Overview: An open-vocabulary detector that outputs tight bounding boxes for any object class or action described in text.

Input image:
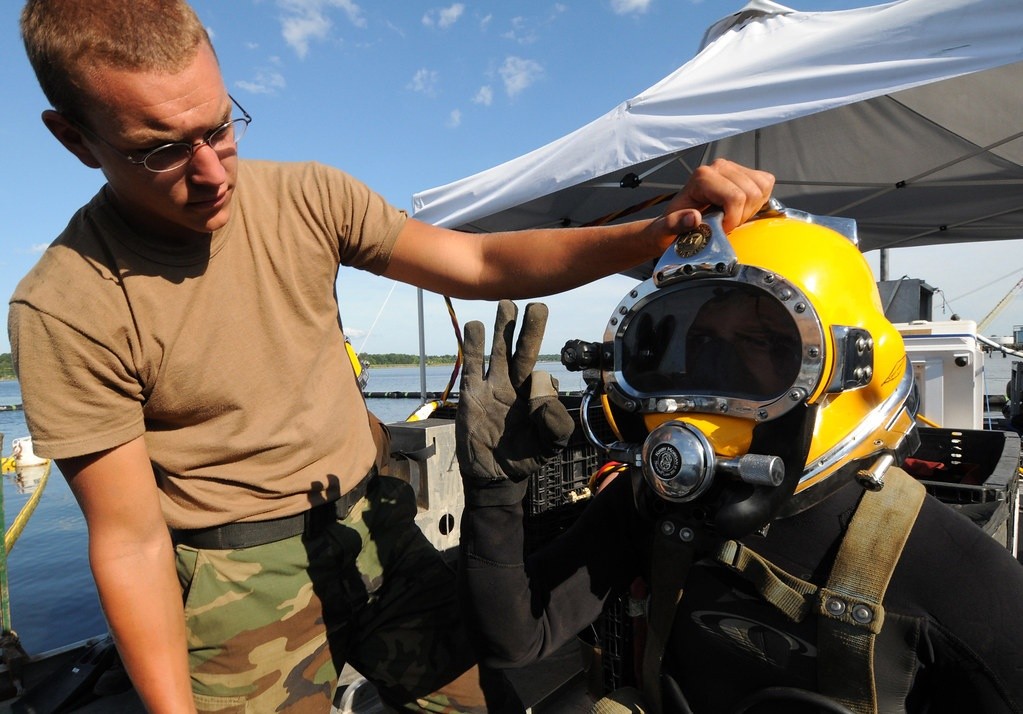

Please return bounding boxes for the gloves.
[456,299,575,508]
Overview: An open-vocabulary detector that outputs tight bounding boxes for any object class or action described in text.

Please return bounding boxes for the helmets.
[600,193,920,538]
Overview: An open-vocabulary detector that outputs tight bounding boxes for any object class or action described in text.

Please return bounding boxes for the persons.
[457,208,1023,714]
[14,454,23,482]
[8,0,778,714]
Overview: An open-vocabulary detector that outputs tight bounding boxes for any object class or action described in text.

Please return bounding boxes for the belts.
[170,465,377,550]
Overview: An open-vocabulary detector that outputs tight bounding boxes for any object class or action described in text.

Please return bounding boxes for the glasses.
[60,93,252,173]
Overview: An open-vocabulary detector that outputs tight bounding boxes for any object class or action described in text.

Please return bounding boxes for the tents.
[415,0,1023,476]
